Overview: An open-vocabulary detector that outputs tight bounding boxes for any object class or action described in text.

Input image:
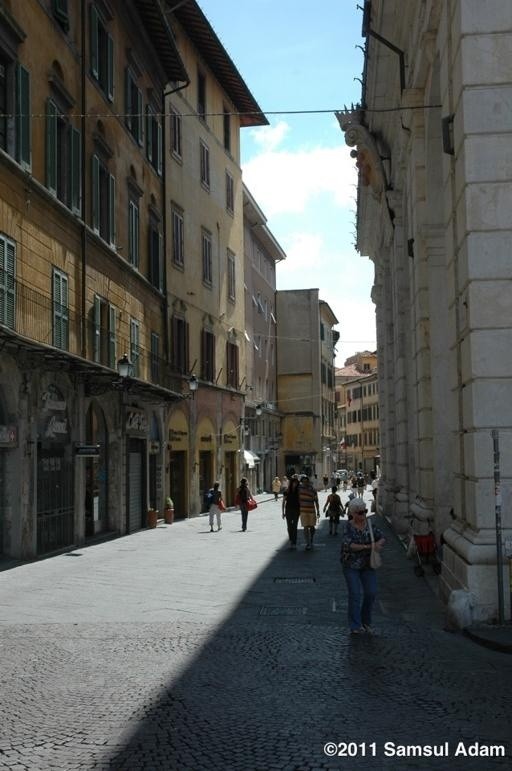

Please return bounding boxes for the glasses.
[355,508,368,516]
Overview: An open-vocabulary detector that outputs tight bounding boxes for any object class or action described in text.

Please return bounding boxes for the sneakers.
[354,625,380,637]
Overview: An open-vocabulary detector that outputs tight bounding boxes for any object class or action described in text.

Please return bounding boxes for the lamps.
[239,405,262,424]
[170,375,200,401]
[84,353,135,399]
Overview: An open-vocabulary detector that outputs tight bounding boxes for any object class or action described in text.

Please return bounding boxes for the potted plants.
[164,497,176,525]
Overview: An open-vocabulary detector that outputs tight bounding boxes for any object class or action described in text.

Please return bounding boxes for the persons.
[234,478,256,531]
[272,470,377,551]
[209,482,224,531]
[341,498,387,635]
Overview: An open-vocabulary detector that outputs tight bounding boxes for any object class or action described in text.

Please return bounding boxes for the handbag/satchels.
[218,499,225,510]
[204,493,211,501]
[370,550,383,569]
[246,499,257,511]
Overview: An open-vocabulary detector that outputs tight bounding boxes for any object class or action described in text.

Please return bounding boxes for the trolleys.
[410,517,443,569]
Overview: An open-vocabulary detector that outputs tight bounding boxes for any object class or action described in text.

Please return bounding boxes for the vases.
[148,509,159,529]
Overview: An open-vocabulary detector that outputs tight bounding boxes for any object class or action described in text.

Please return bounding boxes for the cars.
[334,468,350,481]
